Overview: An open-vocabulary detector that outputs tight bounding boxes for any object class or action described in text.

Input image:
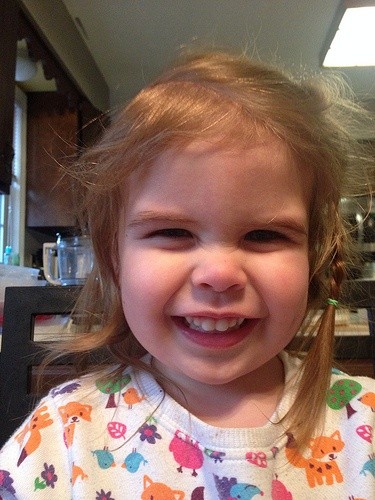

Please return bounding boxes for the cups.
[43,242,59,286]
[46,235,94,285]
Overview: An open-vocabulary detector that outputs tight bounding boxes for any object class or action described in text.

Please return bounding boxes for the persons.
[0,52,375,500]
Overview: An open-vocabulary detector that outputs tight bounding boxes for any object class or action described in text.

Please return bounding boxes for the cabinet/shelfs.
[26,84,99,236]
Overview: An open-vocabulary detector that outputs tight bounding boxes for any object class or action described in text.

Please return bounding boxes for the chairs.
[0,281,375,449]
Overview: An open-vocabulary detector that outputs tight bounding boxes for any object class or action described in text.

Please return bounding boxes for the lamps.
[317,0,375,69]
